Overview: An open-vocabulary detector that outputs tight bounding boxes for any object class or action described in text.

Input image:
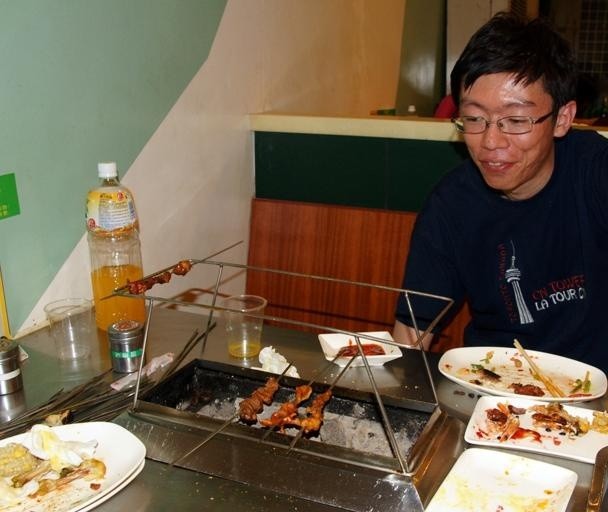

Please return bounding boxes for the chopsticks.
[512,338,567,399]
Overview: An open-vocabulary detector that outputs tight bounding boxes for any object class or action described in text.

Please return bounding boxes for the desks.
[1,303,608,511]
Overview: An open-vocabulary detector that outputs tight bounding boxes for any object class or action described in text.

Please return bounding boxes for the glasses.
[450,107,554,135]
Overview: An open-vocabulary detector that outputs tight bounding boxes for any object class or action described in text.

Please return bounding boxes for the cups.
[219,294,268,359]
[42,296,94,361]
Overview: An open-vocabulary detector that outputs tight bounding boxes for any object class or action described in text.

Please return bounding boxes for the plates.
[0,422,147,512]
[425,448,576,512]
[463,394,608,464]
[317,330,403,367]
[436,345,606,403]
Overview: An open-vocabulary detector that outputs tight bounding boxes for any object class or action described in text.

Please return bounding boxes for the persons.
[396,9,608,380]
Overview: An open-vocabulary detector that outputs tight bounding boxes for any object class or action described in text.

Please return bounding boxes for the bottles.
[106,318,146,374]
[0,339,24,397]
[84,160,147,334]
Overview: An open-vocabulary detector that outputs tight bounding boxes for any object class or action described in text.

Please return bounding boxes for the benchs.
[244,196,471,353]
